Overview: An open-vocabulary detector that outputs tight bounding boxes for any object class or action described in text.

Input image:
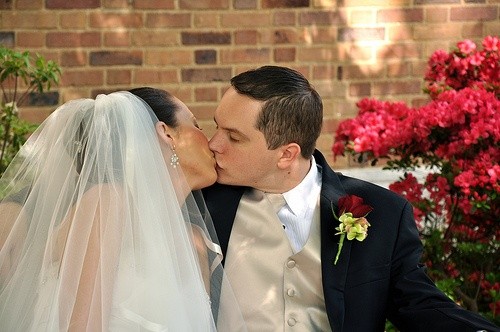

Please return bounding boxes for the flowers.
[330,194,374,267]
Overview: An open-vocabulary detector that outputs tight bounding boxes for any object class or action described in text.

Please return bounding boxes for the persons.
[0,86,218,332]
[1,65,500,332]
